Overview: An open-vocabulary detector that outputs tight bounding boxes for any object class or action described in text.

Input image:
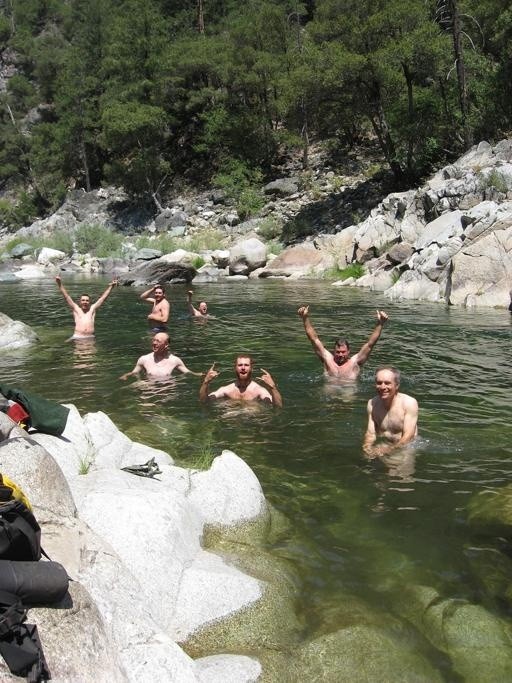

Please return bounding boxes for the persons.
[140,284,170,323]
[188,291,210,324]
[119,332,205,382]
[298,305,388,383]
[362,366,419,458]
[199,355,283,408]
[55,272,118,337]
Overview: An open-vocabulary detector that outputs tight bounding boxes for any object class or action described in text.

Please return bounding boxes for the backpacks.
[0,471,41,561]
